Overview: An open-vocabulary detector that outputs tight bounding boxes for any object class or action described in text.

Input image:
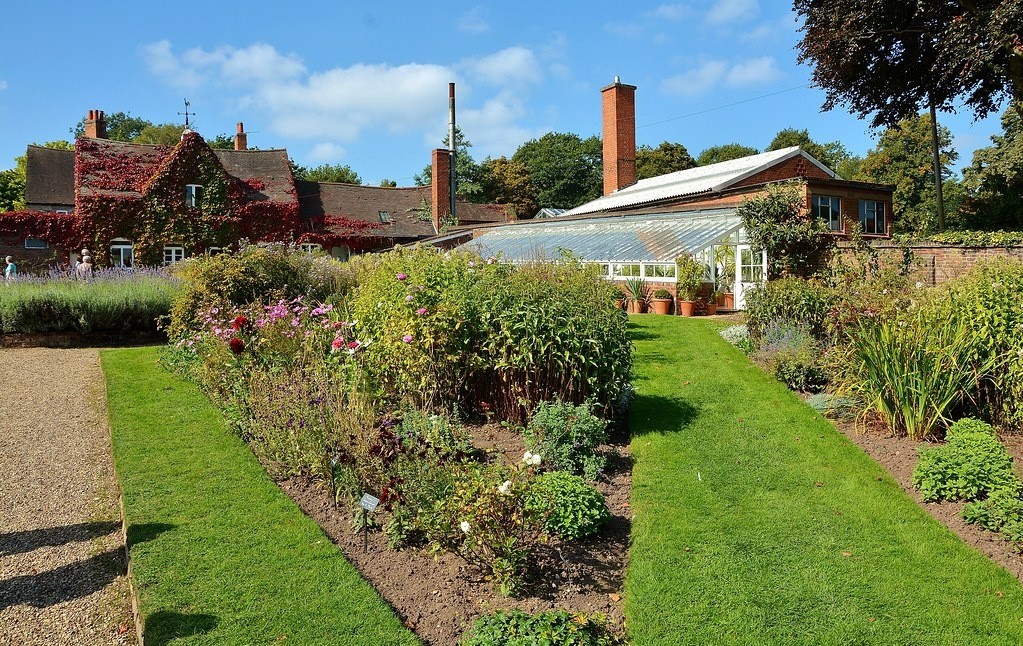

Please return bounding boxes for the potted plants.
[624,277,647,313]
[675,251,703,317]
[706,289,716,314]
[611,288,625,309]
[652,290,672,314]
[723,286,734,308]
[715,290,723,307]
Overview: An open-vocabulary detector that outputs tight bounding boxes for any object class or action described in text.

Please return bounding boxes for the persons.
[78,257,92,278]
[75,248,89,267]
[5,255,16,285]
